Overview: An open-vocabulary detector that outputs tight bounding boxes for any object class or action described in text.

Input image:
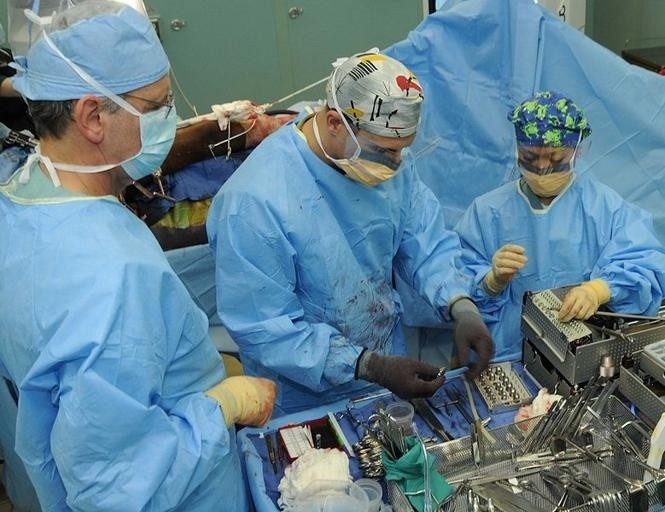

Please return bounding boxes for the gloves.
[447,295,494,380]
[354,347,447,401]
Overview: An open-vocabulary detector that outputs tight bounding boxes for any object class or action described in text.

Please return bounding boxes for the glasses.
[123,91,175,118]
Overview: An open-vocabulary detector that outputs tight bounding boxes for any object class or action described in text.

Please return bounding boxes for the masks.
[516,159,573,198]
[334,146,403,188]
[122,104,178,181]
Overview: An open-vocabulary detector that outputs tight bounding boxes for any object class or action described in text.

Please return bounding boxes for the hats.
[15,0,170,100]
[508,89,592,147]
[328,52,424,135]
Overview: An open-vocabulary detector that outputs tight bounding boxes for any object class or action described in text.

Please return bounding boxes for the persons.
[449,87,665,366]
[0,49,303,207]
[203,48,498,417]
[0,0,277,512]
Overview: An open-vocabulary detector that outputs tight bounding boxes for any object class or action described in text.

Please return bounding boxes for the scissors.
[335,399,369,438]
[351,436,383,478]
